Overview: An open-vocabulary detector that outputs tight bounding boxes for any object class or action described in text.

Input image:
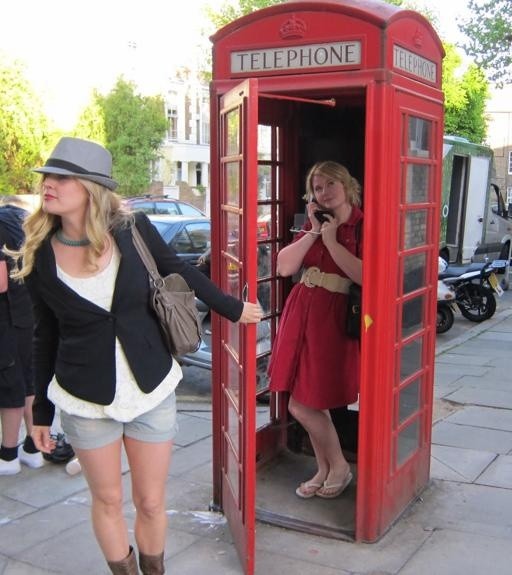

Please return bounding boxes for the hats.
[34,138,119,192]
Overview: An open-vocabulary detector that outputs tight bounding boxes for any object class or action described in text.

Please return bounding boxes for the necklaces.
[56,228,91,247]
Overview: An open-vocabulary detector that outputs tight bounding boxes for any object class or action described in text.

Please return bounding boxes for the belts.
[300,266,352,294]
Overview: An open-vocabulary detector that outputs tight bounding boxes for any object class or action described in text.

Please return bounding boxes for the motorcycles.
[163,247,278,405]
[438,255,509,322]
[403,255,457,334]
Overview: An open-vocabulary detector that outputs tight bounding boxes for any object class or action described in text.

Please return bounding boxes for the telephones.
[311,195,334,225]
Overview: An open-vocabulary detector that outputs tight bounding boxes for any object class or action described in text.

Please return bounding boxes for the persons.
[266,161,363,499]
[0,135,263,575]
[1,204,44,476]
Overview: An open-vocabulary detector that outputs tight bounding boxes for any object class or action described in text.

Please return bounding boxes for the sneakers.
[0,457,22,476]
[18,443,45,469]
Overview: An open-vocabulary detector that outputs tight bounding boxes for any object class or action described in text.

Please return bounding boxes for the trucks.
[404,132,512,274]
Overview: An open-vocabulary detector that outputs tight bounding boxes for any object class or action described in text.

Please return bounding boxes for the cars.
[141,213,274,309]
[121,196,208,222]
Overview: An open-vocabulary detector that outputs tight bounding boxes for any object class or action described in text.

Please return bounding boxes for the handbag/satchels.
[343,283,362,341]
[152,272,203,357]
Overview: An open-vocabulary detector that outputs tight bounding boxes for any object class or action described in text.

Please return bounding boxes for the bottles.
[65,459,81,476]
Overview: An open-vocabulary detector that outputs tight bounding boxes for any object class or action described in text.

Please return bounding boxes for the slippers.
[316,473,353,499]
[296,480,323,499]
